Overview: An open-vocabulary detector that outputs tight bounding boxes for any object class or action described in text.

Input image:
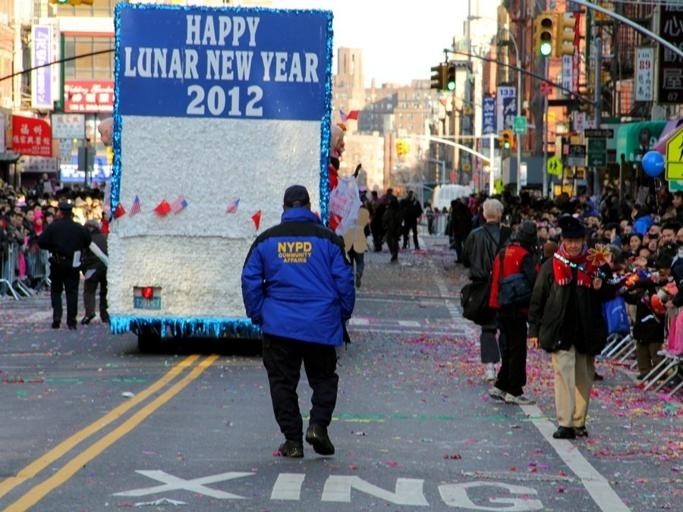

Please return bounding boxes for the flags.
[114,203,126,218]
[129,195,141,217]
[171,194,187,213]
[155,199,170,216]
[95,210,114,232]
[250,209,263,229]
[328,209,343,232]
[227,196,240,215]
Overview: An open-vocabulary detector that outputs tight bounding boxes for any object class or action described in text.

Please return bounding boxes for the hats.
[284,186,310,207]
[86,220,98,230]
[562,220,585,240]
[59,202,74,211]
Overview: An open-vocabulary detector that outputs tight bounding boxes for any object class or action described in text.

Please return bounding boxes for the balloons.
[642,150,665,176]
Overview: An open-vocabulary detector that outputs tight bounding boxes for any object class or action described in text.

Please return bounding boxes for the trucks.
[109,2,333,356]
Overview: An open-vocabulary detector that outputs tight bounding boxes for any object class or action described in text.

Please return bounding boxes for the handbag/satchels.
[496,274,529,305]
[605,299,631,333]
[460,280,496,323]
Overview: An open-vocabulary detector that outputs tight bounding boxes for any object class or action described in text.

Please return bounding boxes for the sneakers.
[486,368,496,380]
[68,323,77,330]
[100,311,110,323]
[505,393,536,405]
[305,424,334,455]
[280,440,304,458]
[575,426,589,437]
[553,426,577,440]
[81,311,95,324]
[52,322,60,329]
[488,387,506,400]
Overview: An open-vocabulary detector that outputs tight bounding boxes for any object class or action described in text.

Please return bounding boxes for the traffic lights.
[535,14,558,59]
[502,131,512,150]
[445,64,456,91]
[430,64,442,93]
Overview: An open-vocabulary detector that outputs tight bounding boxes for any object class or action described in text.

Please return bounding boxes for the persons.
[381,194,402,263]
[379,187,401,239]
[483,221,539,410]
[460,181,513,232]
[460,198,513,380]
[239,183,356,458]
[344,188,366,289]
[35,199,91,330]
[403,190,425,252]
[366,189,387,255]
[613,179,682,380]
[35,172,106,216]
[0,177,34,301]
[525,217,613,438]
[426,199,460,262]
[36,200,91,235]
[514,181,613,263]
[76,216,109,324]
